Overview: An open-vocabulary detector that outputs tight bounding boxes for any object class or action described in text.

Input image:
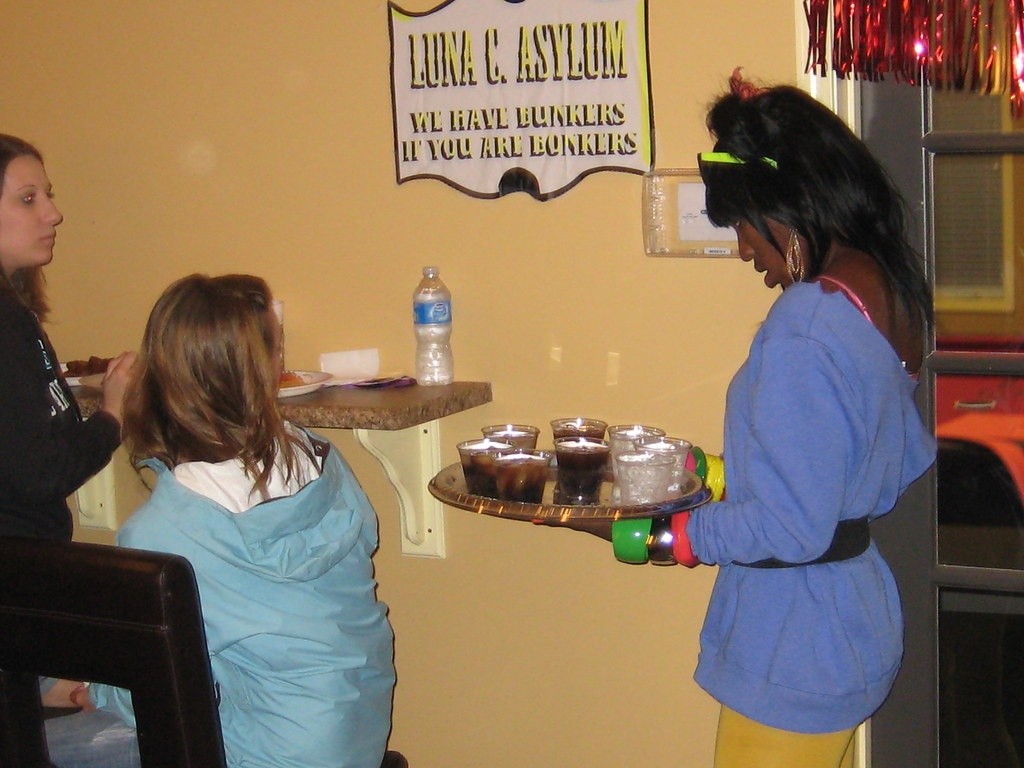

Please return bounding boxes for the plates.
[278,371,335,398]
[59,363,85,386]
[79,372,104,394]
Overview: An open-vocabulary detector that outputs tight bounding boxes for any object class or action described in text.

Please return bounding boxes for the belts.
[732,509,870,570]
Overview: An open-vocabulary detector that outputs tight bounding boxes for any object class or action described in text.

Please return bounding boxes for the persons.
[531,67,939,768]
[69,274,408,768]
[0,133,138,768]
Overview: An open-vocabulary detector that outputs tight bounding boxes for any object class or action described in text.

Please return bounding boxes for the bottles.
[412,266,455,386]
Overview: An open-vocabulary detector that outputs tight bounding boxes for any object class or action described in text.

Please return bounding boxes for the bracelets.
[648,518,679,566]
[671,510,701,568]
[612,518,652,564]
[685,446,725,503]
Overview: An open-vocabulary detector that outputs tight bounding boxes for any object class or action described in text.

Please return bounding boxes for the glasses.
[697,152,778,187]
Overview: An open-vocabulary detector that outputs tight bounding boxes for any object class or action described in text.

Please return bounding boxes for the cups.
[272,301,284,372]
[454,416,693,505]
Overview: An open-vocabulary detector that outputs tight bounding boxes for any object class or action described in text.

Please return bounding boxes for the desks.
[66,381,491,429]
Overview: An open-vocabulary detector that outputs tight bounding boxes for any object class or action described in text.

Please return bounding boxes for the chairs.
[0,535,413,768]
